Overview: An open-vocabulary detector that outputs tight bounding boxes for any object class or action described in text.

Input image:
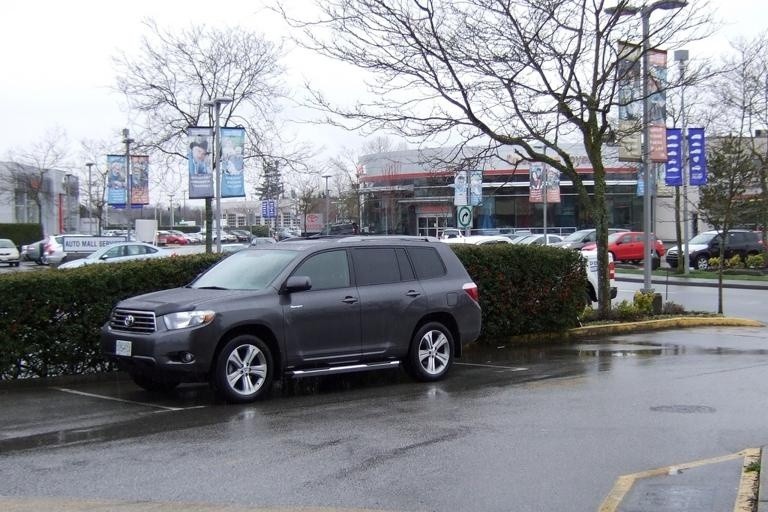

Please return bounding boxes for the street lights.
[168,195,175,228]
[65,173,72,233]
[181,190,187,221]
[203,96,234,252]
[604,0,688,317]
[673,49,690,275]
[85,163,94,235]
[122,128,134,241]
[321,175,332,236]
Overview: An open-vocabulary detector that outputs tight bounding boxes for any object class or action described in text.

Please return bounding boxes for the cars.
[440,229,631,263]
[56,242,173,270]
[360,226,377,234]
[552,196,614,226]
[21,226,298,254]
[0,239,20,266]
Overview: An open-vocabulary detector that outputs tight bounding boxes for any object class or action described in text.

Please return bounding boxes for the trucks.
[179,221,195,227]
[321,223,355,236]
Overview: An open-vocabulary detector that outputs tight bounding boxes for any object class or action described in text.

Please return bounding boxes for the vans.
[667,230,763,271]
[99,235,482,403]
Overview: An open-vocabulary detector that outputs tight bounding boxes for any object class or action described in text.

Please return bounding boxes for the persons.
[456,176,467,193]
[129,165,146,188]
[530,168,543,189]
[107,161,127,190]
[188,137,210,174]
[619,68,640,121]
[227,144,244,174]
[352,222,359,235]
[547,170,559,190]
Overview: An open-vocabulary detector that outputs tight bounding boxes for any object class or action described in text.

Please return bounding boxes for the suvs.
[39,234,93,265]
[581,231,665,266]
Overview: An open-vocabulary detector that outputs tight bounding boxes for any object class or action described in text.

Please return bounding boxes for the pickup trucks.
[439,234,618,316]
